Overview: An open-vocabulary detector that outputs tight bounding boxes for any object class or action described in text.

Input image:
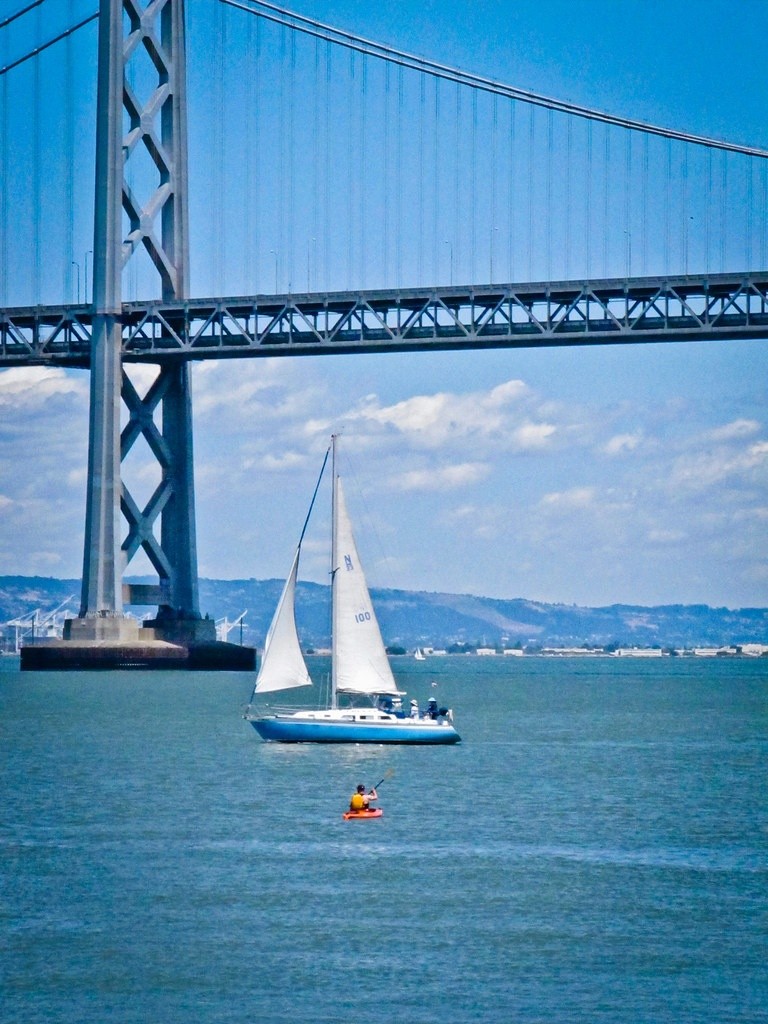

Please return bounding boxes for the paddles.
[349,767,394,807]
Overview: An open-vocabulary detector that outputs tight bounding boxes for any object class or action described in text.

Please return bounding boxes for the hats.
[428,697,435,701]
[410,700,417,705]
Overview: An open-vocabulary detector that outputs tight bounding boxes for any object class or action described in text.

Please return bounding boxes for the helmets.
[357,785,364,790]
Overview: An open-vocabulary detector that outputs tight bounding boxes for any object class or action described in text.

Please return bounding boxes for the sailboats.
[241,430,464,745]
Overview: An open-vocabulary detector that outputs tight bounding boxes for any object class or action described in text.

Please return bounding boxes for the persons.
[410,700,419,717]
[427,697,437,713]
[349,785,378,810]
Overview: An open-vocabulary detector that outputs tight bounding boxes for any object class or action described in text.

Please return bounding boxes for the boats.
[342,807,383,821]
